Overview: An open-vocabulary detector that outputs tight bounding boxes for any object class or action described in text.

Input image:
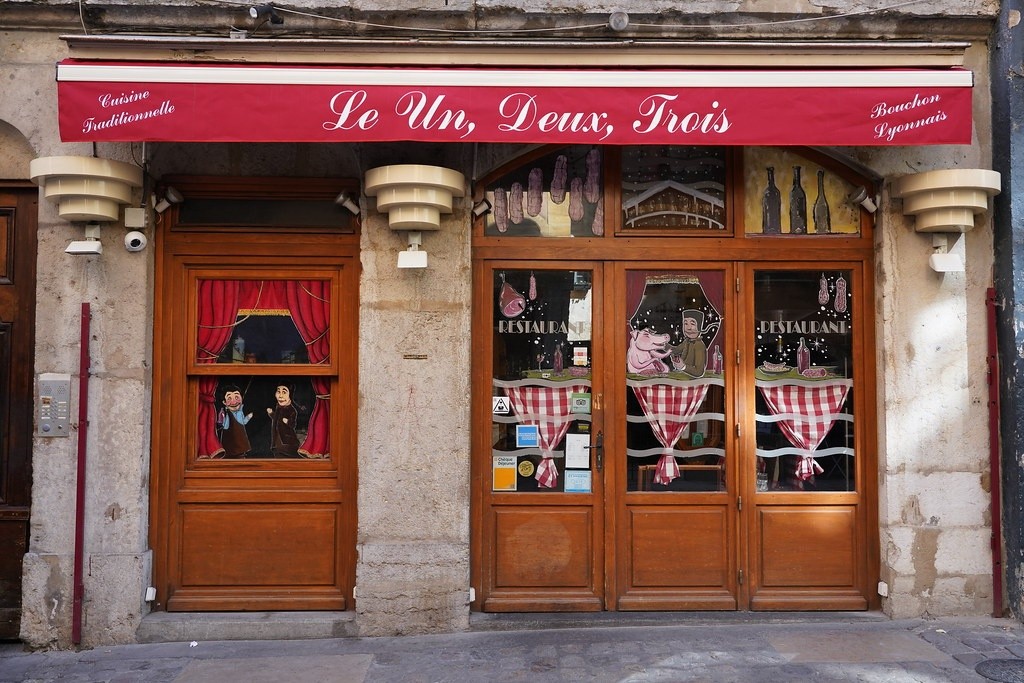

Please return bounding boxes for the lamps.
[853,185,877,215]
[154,187,183,215]
[335,191,359,216]
[250,3,284,24]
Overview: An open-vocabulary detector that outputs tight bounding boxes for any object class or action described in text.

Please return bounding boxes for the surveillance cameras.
[124,231,147,252]
